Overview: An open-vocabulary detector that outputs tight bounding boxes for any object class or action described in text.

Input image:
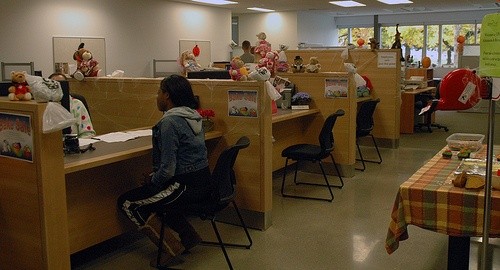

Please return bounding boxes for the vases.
[293,99,310,108]
[202,119,214,131]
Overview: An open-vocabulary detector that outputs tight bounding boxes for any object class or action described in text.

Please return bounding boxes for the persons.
[48,72,96,139]
[117,74,211,268]
[239,41,254,63]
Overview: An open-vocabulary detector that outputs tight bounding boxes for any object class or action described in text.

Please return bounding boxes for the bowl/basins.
[446,133,485,153]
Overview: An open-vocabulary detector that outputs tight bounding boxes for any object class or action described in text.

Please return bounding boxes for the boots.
[164,214,201,254]
[138,212,185,269]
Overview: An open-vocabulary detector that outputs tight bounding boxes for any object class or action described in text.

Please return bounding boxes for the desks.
[399,87,436,135]
[272,108,321,123]
[65,127,222,254]
[385,145,500,270]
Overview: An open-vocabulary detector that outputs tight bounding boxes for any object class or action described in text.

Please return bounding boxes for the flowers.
[198,109,215,120]
[292,92,311,103]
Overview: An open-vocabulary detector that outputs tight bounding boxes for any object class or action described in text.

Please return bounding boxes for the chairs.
[414,93,449,134]
[280,109,345,202]
[159,136,251,270]
[356,98,382,172]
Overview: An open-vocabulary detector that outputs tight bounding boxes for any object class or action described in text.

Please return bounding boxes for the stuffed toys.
[8,71,31,101]
[228,56,255,81]
[290,55,306,73]
[70,43,100,80]
[248,32,289,80]
[305,57,321,73]
[180,50,202,73]
[344,63,369,98]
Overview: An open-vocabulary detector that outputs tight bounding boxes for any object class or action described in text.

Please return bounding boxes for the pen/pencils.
[91,138,100,140]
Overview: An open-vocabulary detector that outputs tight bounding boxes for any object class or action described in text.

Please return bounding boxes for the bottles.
[64,134,79,153]
